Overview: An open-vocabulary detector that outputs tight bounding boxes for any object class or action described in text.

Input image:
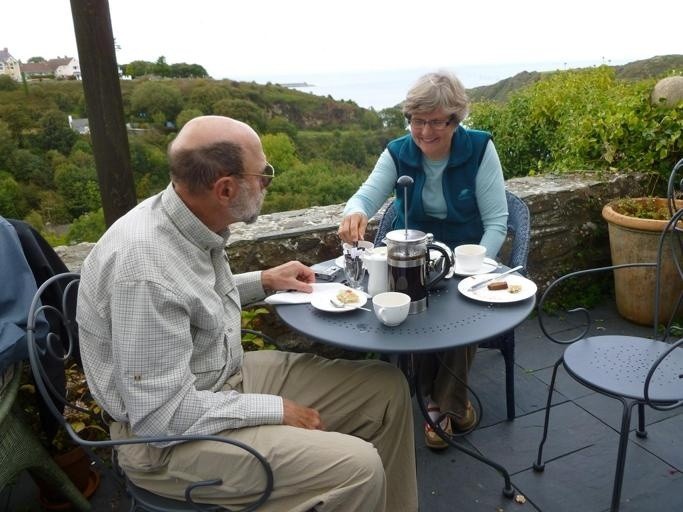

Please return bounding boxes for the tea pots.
[381,229,450,315]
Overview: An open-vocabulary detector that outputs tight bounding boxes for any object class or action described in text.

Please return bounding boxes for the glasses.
[408,117,454,131]
[227,162,274,188]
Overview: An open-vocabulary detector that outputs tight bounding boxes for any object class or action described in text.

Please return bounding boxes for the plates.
[451,257,499,276]
[309,287,367,314]
[430,241,450,262]
[457,273,538,304]
[335,256,366,271]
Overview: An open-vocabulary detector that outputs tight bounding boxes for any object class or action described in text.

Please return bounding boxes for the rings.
[338,227,342,230]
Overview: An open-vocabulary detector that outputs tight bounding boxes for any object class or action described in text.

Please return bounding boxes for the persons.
[335,71,509,452]
[73,113,420,511]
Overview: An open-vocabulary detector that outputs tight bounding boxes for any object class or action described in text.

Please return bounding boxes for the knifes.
[468,265,524,292]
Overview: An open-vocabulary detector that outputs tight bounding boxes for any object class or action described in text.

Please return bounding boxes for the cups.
[372,292,411,327]
[343,240,374,254]
[454,244,486,271]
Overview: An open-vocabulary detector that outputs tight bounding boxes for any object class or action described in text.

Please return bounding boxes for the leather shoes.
[452,400,477,432]
[424,399,453,450]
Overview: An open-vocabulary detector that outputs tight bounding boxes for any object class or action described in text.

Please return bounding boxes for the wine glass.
[343,261,364,292]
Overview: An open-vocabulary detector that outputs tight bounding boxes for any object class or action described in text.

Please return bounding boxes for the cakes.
[337,289,359,304]
